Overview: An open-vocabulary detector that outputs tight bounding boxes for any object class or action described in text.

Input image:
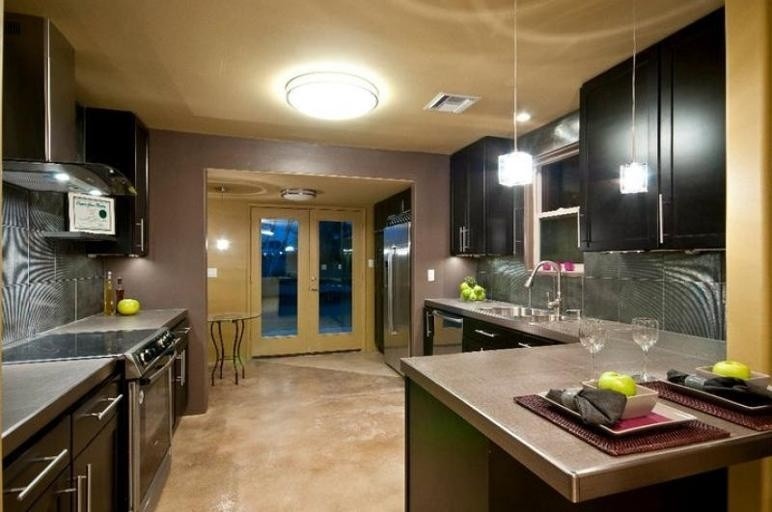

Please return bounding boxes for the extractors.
[3,11,138,199]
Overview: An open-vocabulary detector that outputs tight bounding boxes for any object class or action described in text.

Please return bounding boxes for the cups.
[631,317,659,382]
[579,316,606,382]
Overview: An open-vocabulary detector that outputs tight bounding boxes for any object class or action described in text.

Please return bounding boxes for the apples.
[598,371,636,398]
[712,360,749,382]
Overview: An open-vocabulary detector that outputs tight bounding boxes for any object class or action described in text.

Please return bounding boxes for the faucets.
[524,260,563,314]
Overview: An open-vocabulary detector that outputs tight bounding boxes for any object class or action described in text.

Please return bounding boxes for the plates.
[656,375,771,417]
[536,386,697,437]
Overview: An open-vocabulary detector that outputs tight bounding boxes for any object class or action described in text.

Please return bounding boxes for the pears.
[460,283,487,302]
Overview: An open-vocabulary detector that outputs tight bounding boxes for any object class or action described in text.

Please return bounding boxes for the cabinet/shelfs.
[579,4,726,254]
[65,109,149,258]
[423,297,464,358]
[399,339,772,512]
[89,307,194,437]
[450,135,524,258]
[2,357,125,512]
[518,317,635,348]
[463,303,521,356]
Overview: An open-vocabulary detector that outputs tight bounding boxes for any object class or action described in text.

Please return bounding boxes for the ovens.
[126,349,177,512]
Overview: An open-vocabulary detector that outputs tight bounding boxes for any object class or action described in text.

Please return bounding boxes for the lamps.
[284,71,379,120]
[619,3,648,194]
[280,188,317,201]
[498,0,533,187]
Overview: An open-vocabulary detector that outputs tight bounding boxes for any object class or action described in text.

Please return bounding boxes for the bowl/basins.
[581,380,658,420]
[694,364,770,390]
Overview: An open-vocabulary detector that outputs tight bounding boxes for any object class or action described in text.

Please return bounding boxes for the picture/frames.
[68,192,116,235]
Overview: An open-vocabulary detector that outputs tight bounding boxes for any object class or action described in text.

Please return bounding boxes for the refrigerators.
[379,222,412,380]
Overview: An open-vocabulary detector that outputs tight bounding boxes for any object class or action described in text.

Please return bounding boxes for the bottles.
[115,277,124,313]
[104,271,116,316]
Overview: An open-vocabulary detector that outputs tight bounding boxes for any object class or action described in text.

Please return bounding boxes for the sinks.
[513,316,572,327]
[481,305,551,317]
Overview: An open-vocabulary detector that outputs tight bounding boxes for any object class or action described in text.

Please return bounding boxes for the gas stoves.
[3,326,181,380]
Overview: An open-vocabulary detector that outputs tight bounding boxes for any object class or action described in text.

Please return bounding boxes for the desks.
[208,311,262,386]
